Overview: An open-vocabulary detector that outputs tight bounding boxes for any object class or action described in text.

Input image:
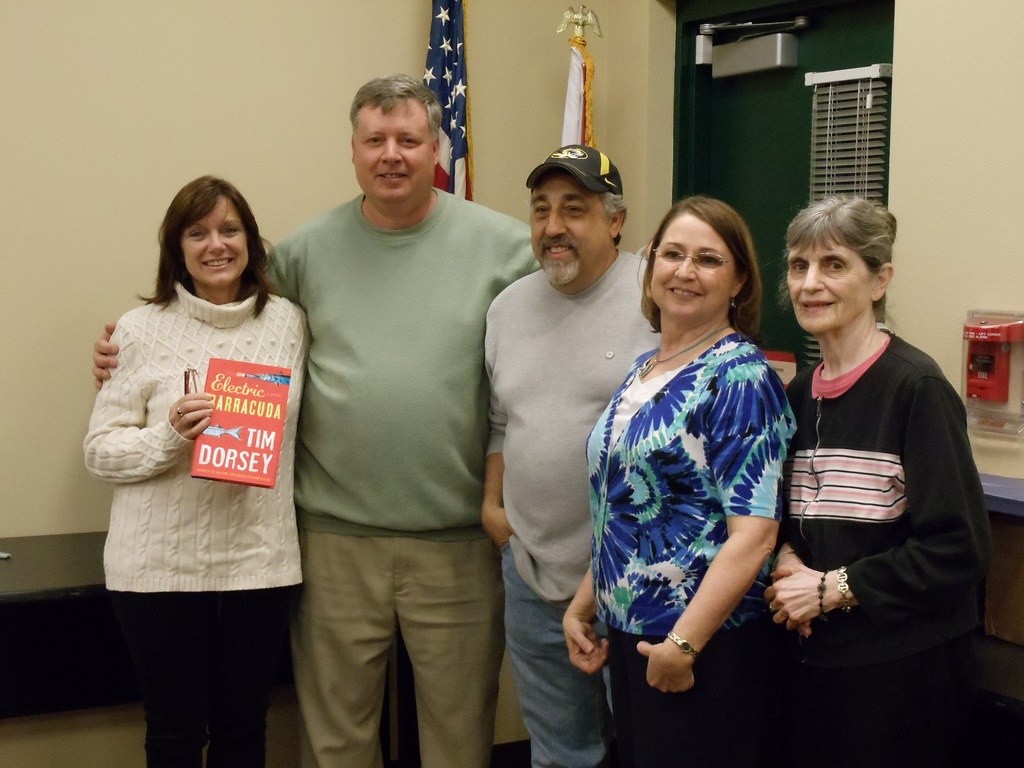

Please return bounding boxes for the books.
[191,358,292,489]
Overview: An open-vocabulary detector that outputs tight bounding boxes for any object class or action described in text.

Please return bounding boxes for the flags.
[561,38,597,150]
[419,0,475,206]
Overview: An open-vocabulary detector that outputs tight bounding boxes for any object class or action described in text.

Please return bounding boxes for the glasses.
[651,249,736,269]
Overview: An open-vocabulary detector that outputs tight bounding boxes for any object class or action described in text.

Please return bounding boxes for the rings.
[770,603,775,613]
[177,408,184,416]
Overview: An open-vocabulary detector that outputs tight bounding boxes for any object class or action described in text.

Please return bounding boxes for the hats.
[525,144,623,201]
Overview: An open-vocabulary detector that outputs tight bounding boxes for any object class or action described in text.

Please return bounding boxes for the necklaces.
[639,326,728,378]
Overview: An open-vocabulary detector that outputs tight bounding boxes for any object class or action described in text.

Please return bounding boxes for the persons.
[478,146,663,768]
[94,72,544,768]
[83,175,314,768]
[763,197,995,768]
[562,195,797,768]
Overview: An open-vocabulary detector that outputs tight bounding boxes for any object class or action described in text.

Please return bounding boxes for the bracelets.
[837,566,851,612]
[773,550,794,566]
[817,571,828,621]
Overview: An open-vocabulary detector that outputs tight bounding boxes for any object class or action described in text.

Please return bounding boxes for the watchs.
[667,631,699,658]
